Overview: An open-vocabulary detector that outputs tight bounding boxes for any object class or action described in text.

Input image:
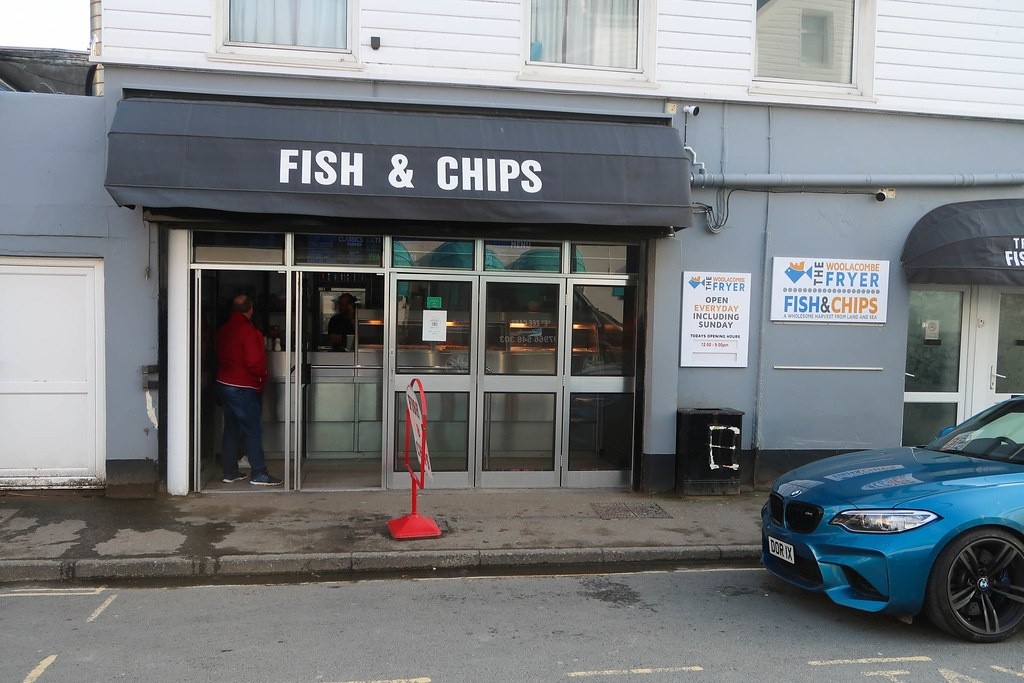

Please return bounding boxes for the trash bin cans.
[676,408,745,496]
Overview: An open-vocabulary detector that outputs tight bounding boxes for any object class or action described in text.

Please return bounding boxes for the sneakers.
[249,473,283,486]
[222,473,248,483]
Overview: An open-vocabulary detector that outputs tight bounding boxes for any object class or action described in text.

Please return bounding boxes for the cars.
[761,394,1024,644]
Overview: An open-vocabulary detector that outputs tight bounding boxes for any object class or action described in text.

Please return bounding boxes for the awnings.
[105,85,694,237]
[902,196,1024,287]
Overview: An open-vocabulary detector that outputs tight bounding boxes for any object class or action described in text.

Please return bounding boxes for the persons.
[326,293,357,343]
[214,295,288,485]
